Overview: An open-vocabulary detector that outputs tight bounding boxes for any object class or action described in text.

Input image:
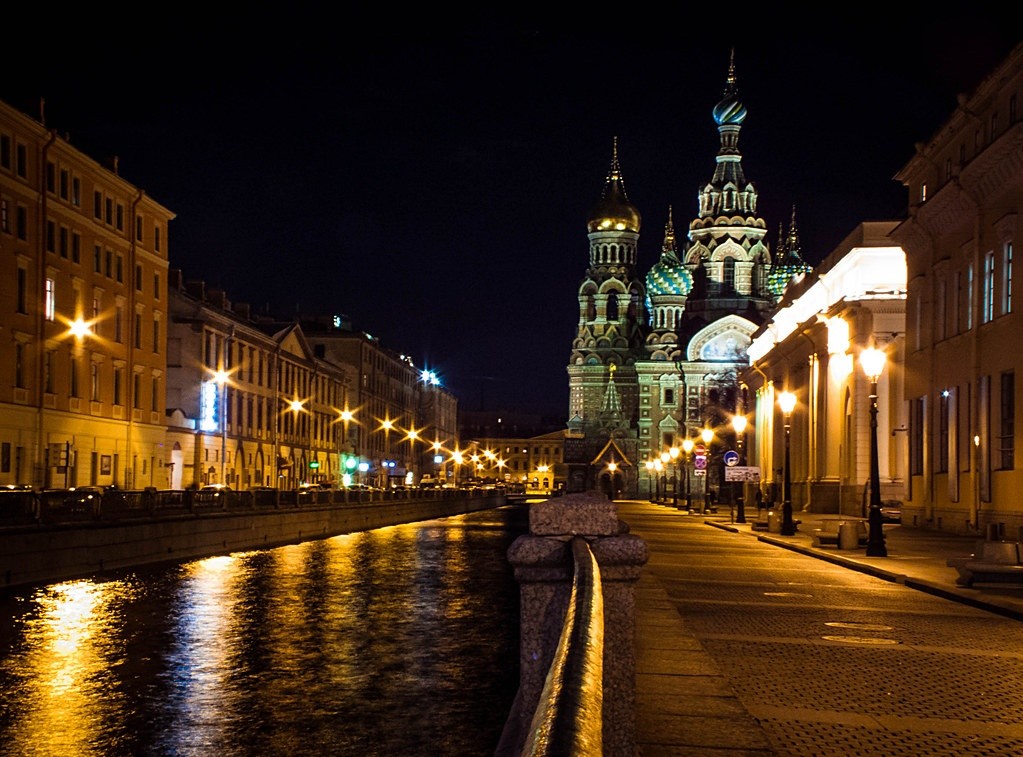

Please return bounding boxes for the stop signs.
[695,445,706,456]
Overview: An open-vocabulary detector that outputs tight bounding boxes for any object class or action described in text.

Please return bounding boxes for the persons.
[755,489,771,511]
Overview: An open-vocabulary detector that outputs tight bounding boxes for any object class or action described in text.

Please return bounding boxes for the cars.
[199,485,238,505]
[65,485,103,512]
[299,478,496,498]
[881,498,903,522]
[0,485,40,520]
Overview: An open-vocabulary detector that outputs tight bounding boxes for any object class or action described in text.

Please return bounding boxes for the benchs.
[807,529,869,549]
[748,519,801,531]
[946,556,1023,587]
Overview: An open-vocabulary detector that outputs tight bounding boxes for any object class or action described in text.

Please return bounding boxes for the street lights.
[646,461,654,502]
[652,459,662,504]
[777,391,799,535]
[669,447,679,507]
[661,452,671,505]
[732,415,748,523]
[700,429,714,514]
[858,346,893,560]
[683,440,695,511]
